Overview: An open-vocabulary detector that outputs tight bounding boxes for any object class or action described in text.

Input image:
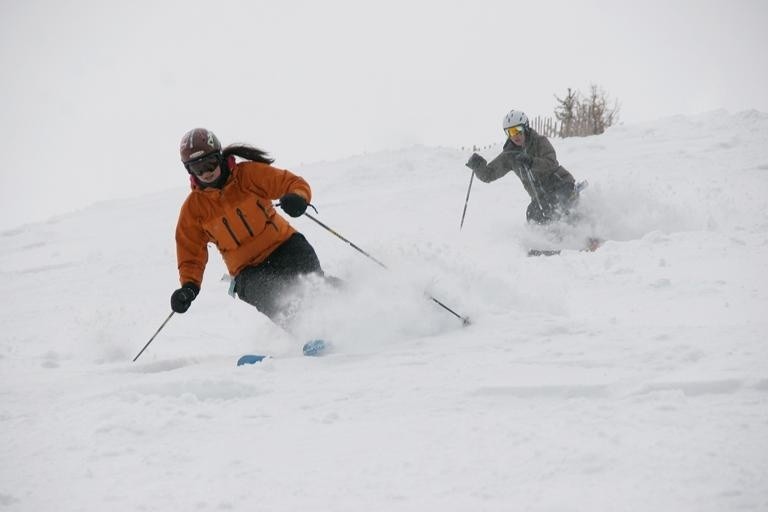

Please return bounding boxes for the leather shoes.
[465,153,487,174]
[171,282,200,313]
[515,151,532,168]
[279,192,307,217]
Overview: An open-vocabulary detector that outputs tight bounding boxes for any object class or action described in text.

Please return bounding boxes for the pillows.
[503,110,529,129]
[180,128,221,164]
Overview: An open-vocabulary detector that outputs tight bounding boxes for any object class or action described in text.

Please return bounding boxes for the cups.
[187,155,222,176]
[505,126,526,136]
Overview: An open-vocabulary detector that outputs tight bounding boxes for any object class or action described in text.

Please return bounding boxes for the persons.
[465,111,575,256]
[170,129,325,336]
